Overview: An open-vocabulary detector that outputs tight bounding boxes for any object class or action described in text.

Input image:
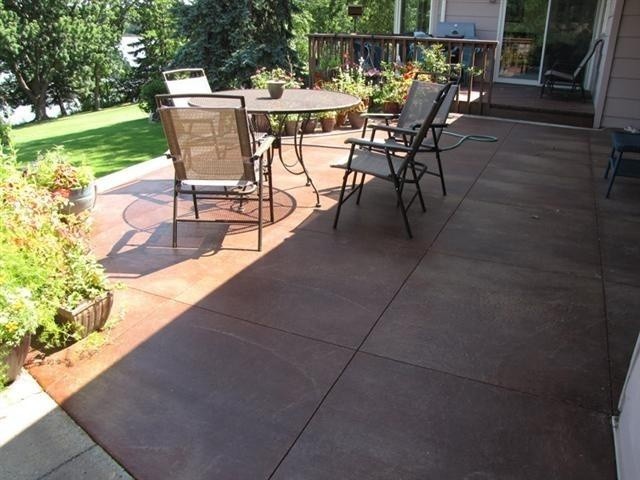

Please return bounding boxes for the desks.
[188,90,361,212]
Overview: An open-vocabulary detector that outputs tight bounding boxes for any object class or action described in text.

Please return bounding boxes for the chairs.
[353,22,476,87]
[540,39,602,103]
[155,94,275,251]
[330,82,455,238]
[604,131,640,198]
[352,80,458,196]
[161,68,268,195]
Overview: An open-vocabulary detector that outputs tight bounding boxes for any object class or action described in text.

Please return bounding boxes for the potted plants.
[373,61,404,113]
[16,225,128,350]
[284,114,303,136]
[0,261,40,385]
[352,83,376,106]
[335,109,350,127]
[348,101,367,128]
[301,113,318,133]
[315,111,337,132]
[25,144,98,225]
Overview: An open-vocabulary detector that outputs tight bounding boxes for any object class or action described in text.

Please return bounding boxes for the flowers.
[249,66,305,90]
[403,61,430,106]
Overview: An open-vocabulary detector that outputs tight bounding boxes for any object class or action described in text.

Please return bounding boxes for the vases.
[253,113,272,135]
